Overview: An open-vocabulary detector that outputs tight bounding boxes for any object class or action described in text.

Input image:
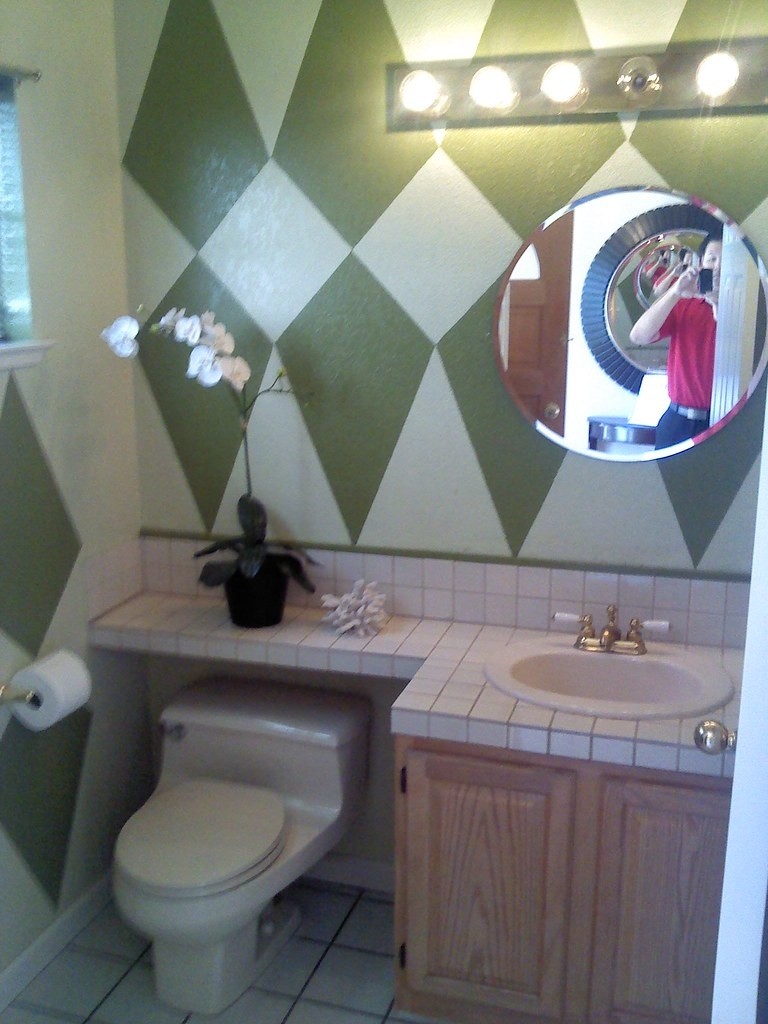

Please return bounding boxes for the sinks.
[482,635,737,720]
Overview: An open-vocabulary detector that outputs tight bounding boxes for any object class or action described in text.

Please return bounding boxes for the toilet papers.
[6,646,93,733]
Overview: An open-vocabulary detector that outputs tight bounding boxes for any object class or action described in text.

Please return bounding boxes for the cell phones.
[699,269,713,294]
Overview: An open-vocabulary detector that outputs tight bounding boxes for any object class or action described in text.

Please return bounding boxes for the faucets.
[551,605,673,656]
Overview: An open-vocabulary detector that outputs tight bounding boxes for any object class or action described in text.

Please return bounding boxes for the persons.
[628,230,722,452]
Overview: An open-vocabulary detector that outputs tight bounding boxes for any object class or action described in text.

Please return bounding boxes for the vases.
[228,564,289,628]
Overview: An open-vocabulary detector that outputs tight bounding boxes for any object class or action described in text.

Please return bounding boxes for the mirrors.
[491,185,768,463]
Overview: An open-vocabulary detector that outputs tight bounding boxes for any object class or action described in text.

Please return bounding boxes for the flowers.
[99,305,323,597]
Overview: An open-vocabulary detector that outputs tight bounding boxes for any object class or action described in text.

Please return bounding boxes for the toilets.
[110,679,374,1014]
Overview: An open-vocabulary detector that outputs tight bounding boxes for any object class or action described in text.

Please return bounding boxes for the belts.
[669,399,710,422]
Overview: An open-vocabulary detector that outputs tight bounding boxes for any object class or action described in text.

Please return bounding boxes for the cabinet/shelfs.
[391,733,732,1024]
